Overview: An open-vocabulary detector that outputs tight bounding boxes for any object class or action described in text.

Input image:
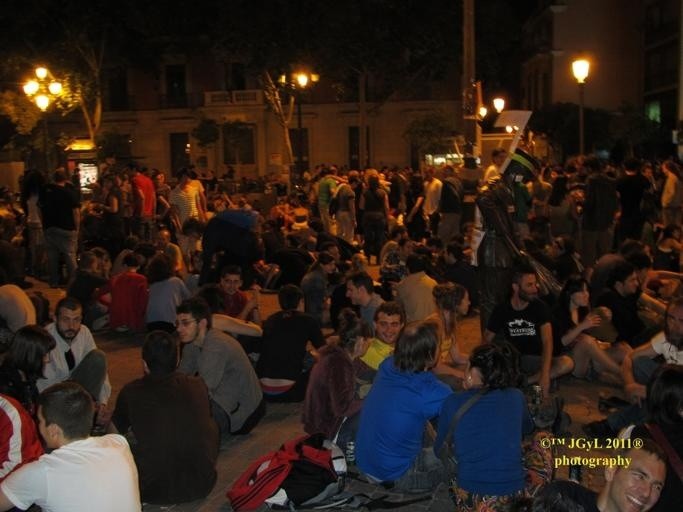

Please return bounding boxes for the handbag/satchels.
[440,443,458,475]
[328,199,340,216]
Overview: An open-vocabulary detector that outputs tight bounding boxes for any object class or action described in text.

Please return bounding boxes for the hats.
[176,167,191,181]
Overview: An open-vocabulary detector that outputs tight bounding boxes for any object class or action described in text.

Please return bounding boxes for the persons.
[0,145,683,403]
[622,363,683,512]
[433,340,557,512]
[353,321,447,502]
[0,381,143,512]
[301,308,365,442]
[105,330,221,503]
[533,438,669,512]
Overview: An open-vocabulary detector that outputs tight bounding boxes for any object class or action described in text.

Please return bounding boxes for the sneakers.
[265,264,282,289]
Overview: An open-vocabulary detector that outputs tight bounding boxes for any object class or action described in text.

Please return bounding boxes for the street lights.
[569,57,590,158]
[23,66,62,178]
[278,71,319,178]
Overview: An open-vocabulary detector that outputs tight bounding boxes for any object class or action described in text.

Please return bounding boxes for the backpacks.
[224,433,347,512]
